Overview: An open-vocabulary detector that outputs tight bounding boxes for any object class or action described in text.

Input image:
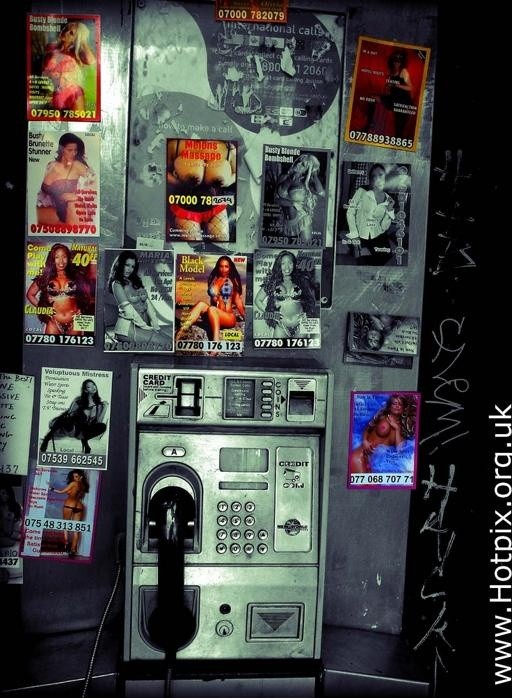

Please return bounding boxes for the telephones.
[148,486,196,648]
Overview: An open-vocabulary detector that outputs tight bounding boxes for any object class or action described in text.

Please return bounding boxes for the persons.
[351,395,416,472]
[41,23,96,118]
[39,133,97,225]
[361,46,415,139]
[46,470,86,556]
[166,140,236,241]
[273,154,327,246]
[346,166,397,265]
[254,250,319,340]
[106,253,171,346]
[0,486,23,583]
[179,257,244,355]
[40,379,106,455]
[355,314,392,352]
[27,244,93,336]
